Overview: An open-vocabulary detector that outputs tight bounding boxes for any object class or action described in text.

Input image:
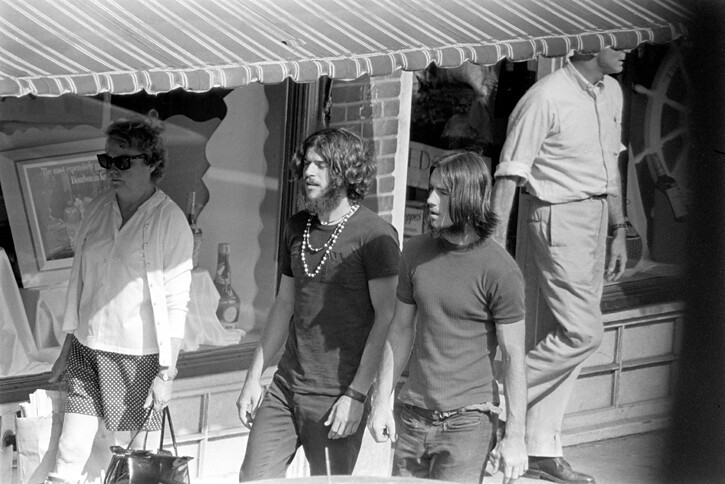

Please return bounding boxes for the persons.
[367,149,530,484]
[236,129,403,484]
[44,119,194,484]
[486,47,631,484]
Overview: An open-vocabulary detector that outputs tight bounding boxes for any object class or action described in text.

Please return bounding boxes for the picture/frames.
[0,137,108,288]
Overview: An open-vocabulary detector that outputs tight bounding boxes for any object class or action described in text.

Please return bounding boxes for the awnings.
[0,0,697,99]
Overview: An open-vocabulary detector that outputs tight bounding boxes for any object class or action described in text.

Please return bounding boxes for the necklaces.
[301,200,358,278]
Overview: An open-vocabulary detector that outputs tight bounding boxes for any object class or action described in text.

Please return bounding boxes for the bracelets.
[344,387,366,403]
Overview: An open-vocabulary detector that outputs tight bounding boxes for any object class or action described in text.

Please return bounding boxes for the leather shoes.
[522,454,597,484]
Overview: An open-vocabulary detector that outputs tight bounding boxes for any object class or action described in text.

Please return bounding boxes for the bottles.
[184,191,202,271]
[212,243,241,330]
[64,189,82,254]
[649,152,689,221]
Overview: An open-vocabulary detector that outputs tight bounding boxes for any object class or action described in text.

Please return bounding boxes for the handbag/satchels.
[14,388,116,484]
[103,403,195,484]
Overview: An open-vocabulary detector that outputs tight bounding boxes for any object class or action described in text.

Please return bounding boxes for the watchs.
[611,223,628,231]
[157,373,175,381]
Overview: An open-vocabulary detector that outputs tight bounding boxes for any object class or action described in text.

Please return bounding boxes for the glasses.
[96,153,146,170]
